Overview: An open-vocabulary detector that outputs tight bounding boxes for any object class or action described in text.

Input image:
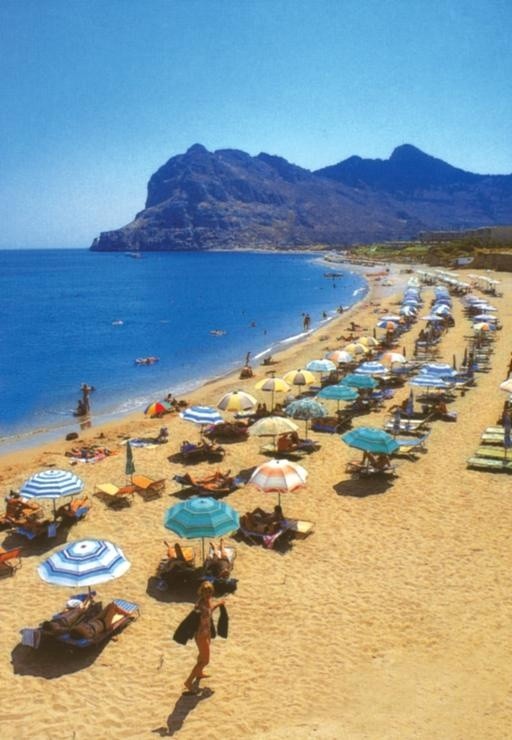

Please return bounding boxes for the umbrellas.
[125,440,138,490]
[16,469,85,517]
[163,495,243,568]
[36,537,133,604]
[245,458,310,506]
[143,400,172,419]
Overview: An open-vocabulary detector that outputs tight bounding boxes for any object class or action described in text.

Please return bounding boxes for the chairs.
[162,541,248,590]
[172,437,243,500]
[259,435,322,462]
[341,408,439,481]
[93,480,138,509]
[237,505,317,551]
[0,495,92,577]
[129,473,167,502]
[18,590,146,649]
[467,424,511,473]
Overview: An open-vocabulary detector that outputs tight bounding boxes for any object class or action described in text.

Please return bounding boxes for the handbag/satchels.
[173,610,200,645]
[190,617,216,639]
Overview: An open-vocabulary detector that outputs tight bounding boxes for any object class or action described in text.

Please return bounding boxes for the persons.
[72,399,88,417]
[201,467,232,493]
[9,490,40,512]
[161,540,191,592]
[40,589,97,630]
[65,443,112,460]
[134,356,161,365]
[237,512,280,535]
[182,580,228,692]
[246,503,286,523]
[52,496,90,521]
[165,393,188,413]
[303,304,344,330]
[23,516,52,531]
[68,601,134,640]
[79,383,95,411]
[208,537,231,579]
